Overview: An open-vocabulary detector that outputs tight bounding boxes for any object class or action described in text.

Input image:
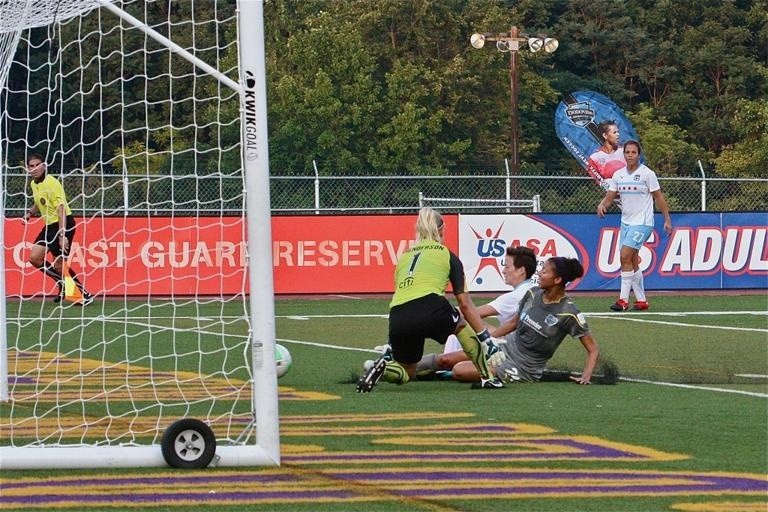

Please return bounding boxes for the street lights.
[470,26,560,213]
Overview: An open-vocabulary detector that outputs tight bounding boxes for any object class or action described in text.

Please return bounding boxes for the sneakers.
[610,299,648,311]
[54,286,65,302]
[479,377,504,388]
[82,292,91,303]
[356,344,393,394]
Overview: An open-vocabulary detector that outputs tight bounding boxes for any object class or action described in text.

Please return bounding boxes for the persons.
[366,246,541,379]
[25,157,93,308]
[418,257,601,384]
[597,140,670,311]
[356,203,508,393]
[587,119,630,183]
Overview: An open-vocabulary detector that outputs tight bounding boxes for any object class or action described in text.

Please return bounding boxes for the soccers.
[276,344,292,378]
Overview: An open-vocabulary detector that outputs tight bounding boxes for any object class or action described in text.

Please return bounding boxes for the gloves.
[476,329,506,367]
